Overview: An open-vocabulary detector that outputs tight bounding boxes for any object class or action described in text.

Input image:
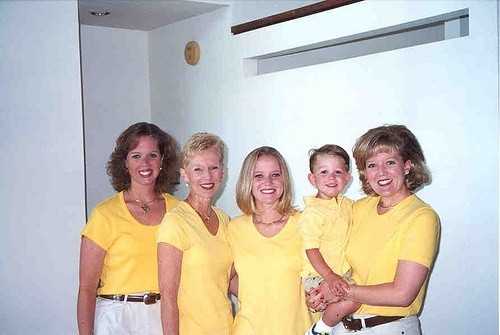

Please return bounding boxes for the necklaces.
[185,197,216,223]
[377,193,413,209]
[252,211,285,225]
[127,190,158,213]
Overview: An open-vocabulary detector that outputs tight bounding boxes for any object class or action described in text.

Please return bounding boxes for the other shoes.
[305,323,332,335]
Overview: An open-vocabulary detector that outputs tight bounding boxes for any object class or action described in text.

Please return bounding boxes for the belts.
[96,291,160,305]
[341,315,405,332]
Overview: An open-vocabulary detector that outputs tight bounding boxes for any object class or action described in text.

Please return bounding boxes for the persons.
[299,143,361,335]
[76,121,181,335]
[305,121,441,335]
[228,146,315,335]
[157,128,239,335]
